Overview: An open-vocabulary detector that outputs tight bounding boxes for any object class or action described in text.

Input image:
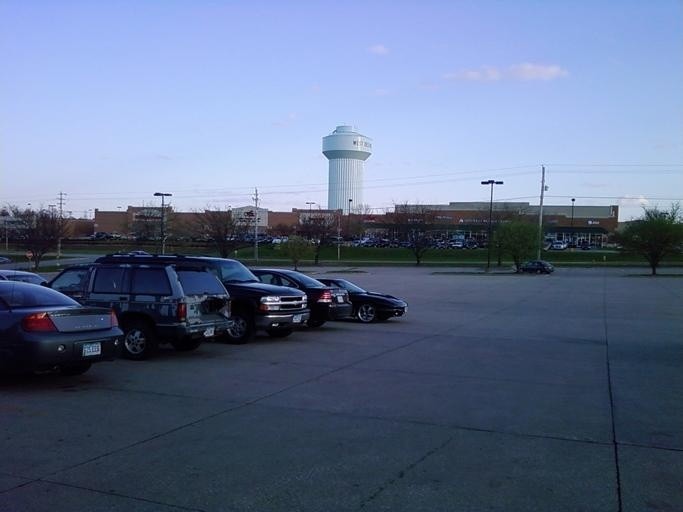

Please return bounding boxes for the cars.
[518,260,554,274]
[0,268,126,388]
[90,231,237,241]
[0,257,12,263]
[246,233,494,249]
[582,242,591,250]
[250,266,409,330]
[552,240,569,250]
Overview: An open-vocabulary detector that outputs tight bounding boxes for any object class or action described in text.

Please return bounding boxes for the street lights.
[306,202,316,226]
[153,192,172,254]
[481,179,504,269]
[348,199,353,232]
[571,198,575,225]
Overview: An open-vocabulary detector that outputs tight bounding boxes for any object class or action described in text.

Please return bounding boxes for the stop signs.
[25,250,33,260]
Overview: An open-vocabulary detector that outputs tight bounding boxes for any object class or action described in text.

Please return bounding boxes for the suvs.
[40,249,313,362]
